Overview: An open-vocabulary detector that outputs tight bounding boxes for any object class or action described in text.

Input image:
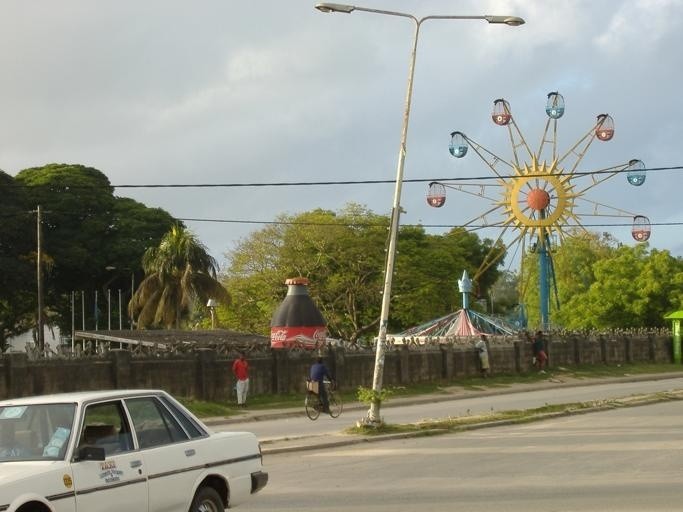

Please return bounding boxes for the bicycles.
[304,376,343,420]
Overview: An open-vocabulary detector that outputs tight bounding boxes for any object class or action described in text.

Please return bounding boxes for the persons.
[232,351,250,408]
[310,357,335,413]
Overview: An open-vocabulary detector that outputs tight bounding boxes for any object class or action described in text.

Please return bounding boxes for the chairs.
[1,420,165,458]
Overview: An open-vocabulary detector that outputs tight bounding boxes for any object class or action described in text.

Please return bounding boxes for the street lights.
[104,267,135,330]
[205,297,217,328]
[313,1,526,425]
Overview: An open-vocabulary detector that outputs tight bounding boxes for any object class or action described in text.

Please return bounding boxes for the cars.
[0,390,269,512]
